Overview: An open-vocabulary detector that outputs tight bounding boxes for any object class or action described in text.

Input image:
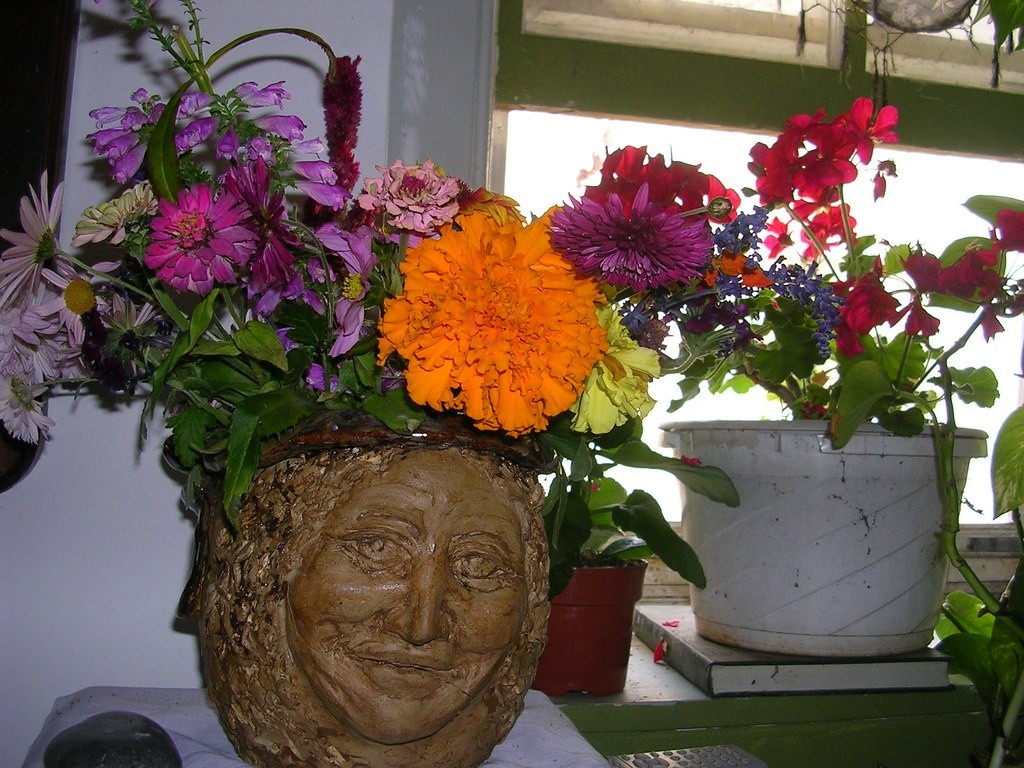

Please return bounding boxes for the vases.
[523,558,649,696]
[659,420,989,663]
[156,396,554,768]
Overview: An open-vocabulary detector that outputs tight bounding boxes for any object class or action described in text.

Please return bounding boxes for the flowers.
[0,0,1024,461]
